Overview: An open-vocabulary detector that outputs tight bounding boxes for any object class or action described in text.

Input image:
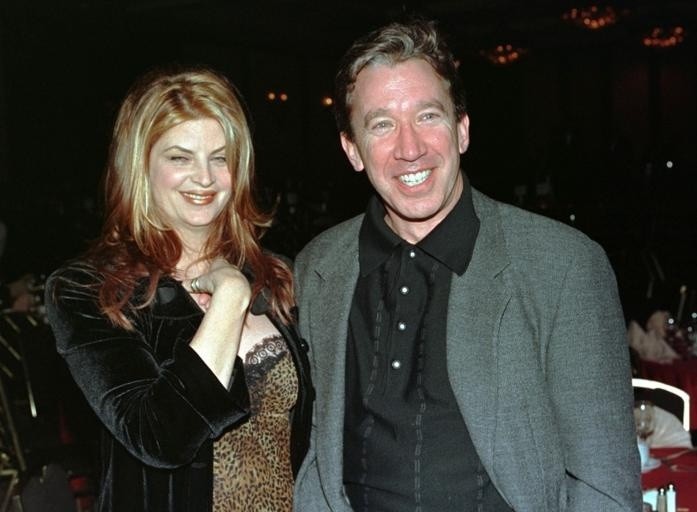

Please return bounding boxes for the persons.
[278,18,646,511]
[43,58,304,512]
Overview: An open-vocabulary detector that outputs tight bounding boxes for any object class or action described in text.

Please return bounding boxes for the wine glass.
[663,316,680,348]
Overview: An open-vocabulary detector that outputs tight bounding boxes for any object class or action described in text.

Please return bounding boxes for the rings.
[190,278,194,292]
[194,277,201,294]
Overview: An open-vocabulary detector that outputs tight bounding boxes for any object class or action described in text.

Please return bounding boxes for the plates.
[641,459,661,474]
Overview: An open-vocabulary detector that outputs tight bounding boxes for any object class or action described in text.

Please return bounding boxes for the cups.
[633,399,653,465]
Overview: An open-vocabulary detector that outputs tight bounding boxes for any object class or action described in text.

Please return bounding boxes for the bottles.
[657,483,677,511]
